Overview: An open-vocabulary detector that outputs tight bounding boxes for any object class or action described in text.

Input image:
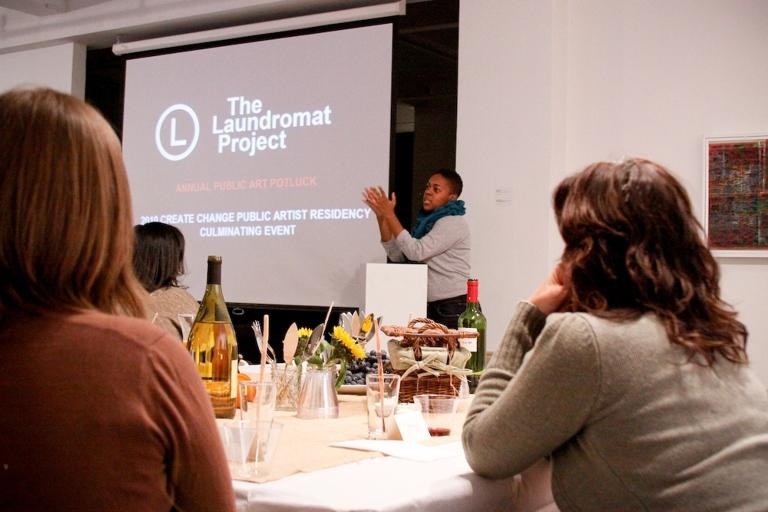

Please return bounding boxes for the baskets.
[392,377,461,402]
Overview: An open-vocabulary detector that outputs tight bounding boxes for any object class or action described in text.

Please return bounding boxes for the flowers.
[287,321,365,389]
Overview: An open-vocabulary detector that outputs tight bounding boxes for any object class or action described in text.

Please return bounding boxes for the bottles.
[456,276,487,397]
[186,254,240,420]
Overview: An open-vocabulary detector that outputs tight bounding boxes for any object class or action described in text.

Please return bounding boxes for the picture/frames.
[702,133,768,259]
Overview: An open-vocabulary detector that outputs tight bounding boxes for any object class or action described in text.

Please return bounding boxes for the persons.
[361,168,482,329]
[462,158,768,512]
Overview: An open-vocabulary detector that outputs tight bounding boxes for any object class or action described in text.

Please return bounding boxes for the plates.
[336,382,390,395]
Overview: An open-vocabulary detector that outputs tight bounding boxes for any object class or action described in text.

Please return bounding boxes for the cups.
[239,380,277,475]
[269,362,303,413]
[365,372,401,441]
[413,391,461,438]
